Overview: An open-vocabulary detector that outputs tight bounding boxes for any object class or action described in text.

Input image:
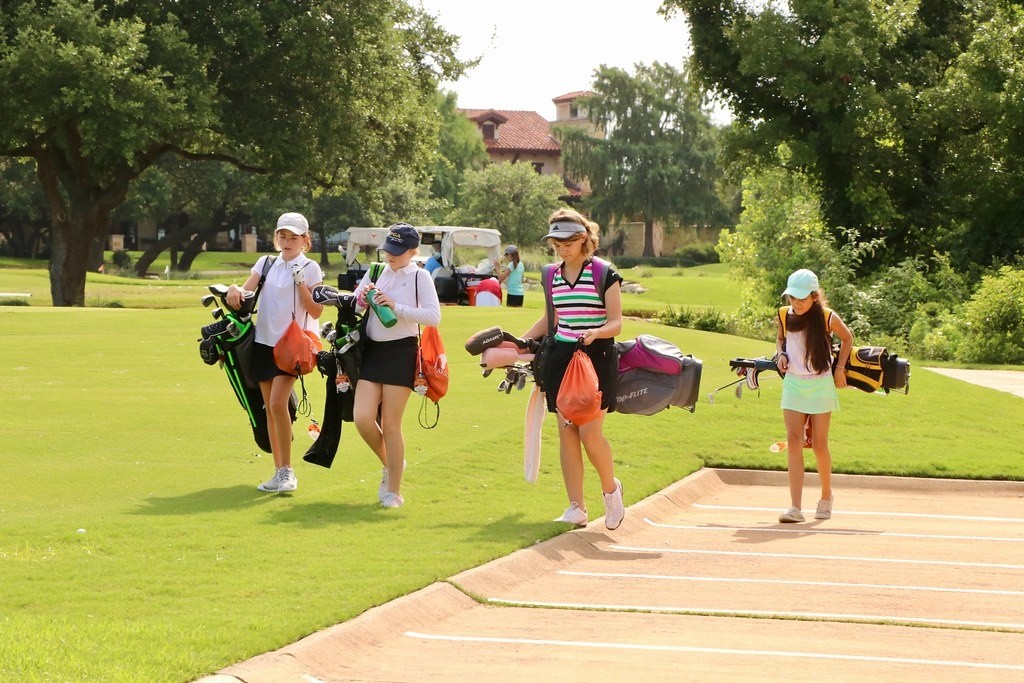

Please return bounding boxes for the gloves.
[290,264,304,285]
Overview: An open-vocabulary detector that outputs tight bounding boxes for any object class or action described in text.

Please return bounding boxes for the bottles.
[367,287,398,328]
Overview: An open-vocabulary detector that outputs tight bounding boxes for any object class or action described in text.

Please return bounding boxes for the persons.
[518,207,626,531]
[417,241,443,276]
[353,222,440,509]
[776,269,853,523]
[495,245,524,307]
[227,212,323,492]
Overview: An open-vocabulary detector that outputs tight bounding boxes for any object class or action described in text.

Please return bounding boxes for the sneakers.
[779,508,805,522]
[553,503,587,527]
[815,493,834,519]
[379,458,406,498]
[256,469,278,493]
[384,491,403,507]
[277,465,297,493]
[601,478,624,530]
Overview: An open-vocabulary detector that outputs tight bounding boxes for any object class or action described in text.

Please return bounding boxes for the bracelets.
[836,365,845,369]
[778,352,789,364]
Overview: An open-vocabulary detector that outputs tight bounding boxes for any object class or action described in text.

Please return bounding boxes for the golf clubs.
[237,290,255,323]
[201,294,219,308]
[211,308,226,320]
[736,375,780,399]
[730,357,756,376]
[338,330,360,355]
[708,376,747,406]
[319,321,337,343]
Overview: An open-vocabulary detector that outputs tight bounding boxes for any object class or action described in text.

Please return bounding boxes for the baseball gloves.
[481,363,536,394]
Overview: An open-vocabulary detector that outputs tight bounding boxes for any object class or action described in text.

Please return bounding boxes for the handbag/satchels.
[415,324,449,402]
[273,318,322,375]
[554,339,608,427]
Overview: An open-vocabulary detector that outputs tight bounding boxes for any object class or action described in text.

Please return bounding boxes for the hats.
[779,269,818,299]
[377,222,419,256]
[540,222,587,241]
[275,212,309,235]
[503,245,517,256]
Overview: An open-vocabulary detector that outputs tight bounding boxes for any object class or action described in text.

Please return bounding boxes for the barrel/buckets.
[466,286,478,305]
